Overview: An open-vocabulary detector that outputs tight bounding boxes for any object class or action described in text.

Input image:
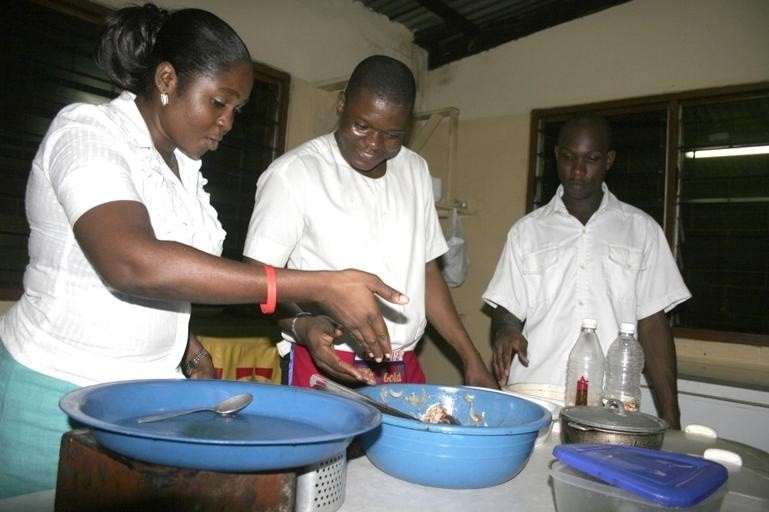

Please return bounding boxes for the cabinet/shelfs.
[564,319,606,407]
[601,322,646,411]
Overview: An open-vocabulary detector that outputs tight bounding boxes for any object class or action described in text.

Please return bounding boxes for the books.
[546,436,731,512]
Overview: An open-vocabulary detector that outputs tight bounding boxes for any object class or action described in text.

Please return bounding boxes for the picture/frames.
[0,427,769,512]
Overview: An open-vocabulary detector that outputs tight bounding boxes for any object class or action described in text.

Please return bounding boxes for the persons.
[0,1,410,497]
[481,113,693,431]
[243,54,500,391]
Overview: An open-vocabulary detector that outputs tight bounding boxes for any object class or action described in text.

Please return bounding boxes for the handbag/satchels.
[260,264,277,314]
[185,349,210,376]
[291,311,311,345]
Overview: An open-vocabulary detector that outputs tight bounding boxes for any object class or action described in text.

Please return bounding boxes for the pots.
[560,398,665,449]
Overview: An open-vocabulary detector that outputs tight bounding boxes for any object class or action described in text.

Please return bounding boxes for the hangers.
[136,390,254,426]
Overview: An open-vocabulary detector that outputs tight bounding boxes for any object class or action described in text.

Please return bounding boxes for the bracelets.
[349,382,553,490]
